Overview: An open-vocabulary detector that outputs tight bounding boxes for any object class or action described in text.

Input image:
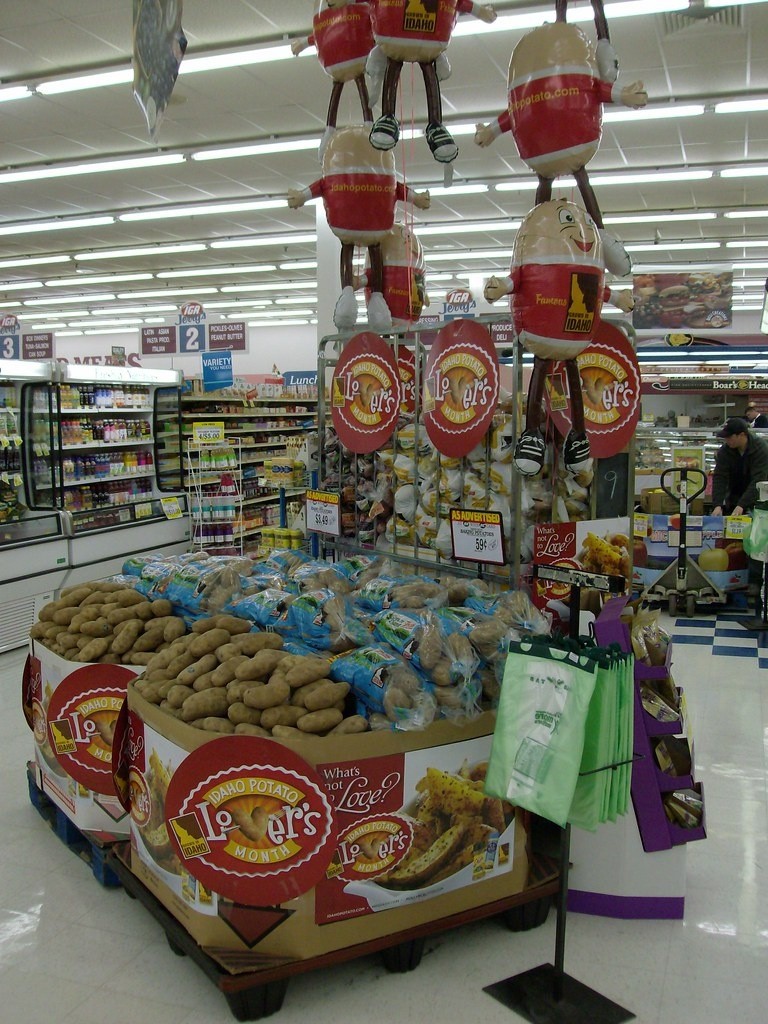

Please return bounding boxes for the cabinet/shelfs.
[153,396,332,555]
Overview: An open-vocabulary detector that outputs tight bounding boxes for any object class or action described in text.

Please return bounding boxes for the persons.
[474,21,648,277]
[711,417,768,597]
[744,407,768,428]
[291,0,498,164]
[555,0,619,83]
[288,127,430,347]
[484,199,635,475]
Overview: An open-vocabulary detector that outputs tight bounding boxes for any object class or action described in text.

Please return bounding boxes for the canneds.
[260,528,302,551]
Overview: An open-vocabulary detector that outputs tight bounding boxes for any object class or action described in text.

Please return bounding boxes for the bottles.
[0,378,23,472]
[30,449,157,485]
[34,416,154,446]
[32,384,152,414]
[192,442,237,542]
[37,479,159,532]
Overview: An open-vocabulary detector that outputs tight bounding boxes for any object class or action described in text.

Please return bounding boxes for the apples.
[611,533,647,567]
[698,540,751,571]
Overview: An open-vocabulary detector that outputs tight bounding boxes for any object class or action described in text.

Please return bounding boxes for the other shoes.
[748,584,758,596]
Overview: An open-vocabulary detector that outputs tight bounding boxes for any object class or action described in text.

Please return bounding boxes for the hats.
[717,417,748,438]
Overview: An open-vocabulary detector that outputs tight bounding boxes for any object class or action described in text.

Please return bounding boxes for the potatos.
[138,748,184,874]
[371,763,517,890]
[30,546,553,738]
[232,806,268,842]
[94,720,118,746]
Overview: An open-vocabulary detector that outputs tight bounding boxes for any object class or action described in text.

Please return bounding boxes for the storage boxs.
[631,504,750,593]
[128,675,530,977]
[641,486,703,513]
[28,634,149,844]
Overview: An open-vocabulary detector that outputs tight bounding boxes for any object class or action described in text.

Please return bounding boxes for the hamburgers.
[659,285,690,307]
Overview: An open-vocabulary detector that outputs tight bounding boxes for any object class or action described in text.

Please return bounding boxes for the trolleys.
[639,465,731,617]
